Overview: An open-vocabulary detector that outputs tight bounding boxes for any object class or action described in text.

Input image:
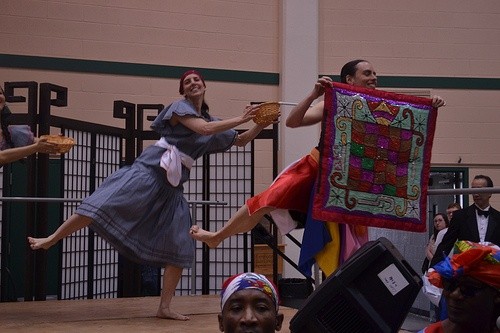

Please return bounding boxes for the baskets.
[246,102,280,128]
[39,134,75,153]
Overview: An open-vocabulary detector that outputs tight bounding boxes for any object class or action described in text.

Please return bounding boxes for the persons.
[189,60,446,278]
[218,271,284,333]
[27,71,282,321]
[422,203,461,322]
[429,175,500,268]
[0,87,61,167]
[417,240,500,333]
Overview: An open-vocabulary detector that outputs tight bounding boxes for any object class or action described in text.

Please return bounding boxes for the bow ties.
[474,204,490,218]
